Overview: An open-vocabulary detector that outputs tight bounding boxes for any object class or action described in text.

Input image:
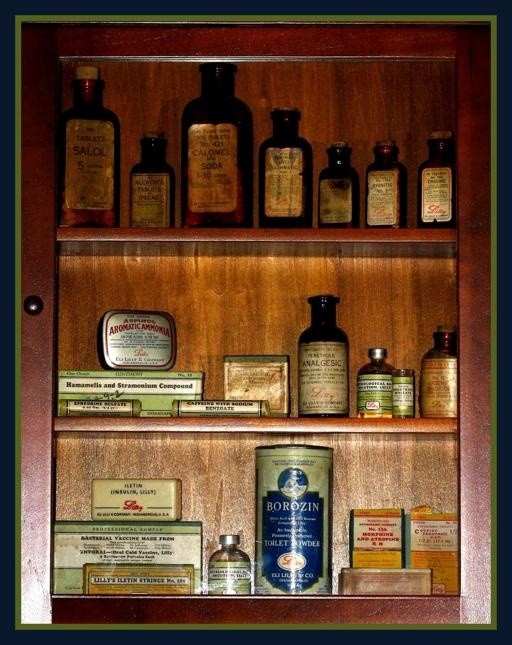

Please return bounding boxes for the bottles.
[127,129,177,228]
[209,535,251,598]
[365,139,407,227]
[317,141,361,226]
[256,105,313,227]
[415,130,457,225]
[296,293,458,417]
[180,59,255,226]
[56,63,122,227]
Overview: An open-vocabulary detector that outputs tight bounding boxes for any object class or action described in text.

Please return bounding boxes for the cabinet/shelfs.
[8,8,501,633]
